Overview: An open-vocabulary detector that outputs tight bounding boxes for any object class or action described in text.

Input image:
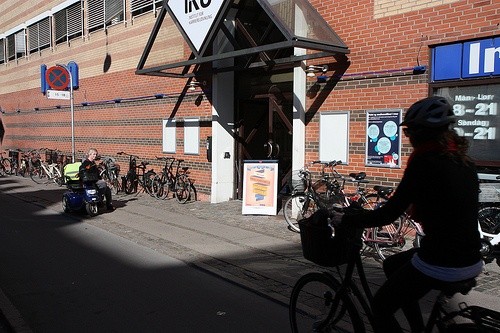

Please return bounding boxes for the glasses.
[403,128,414,137]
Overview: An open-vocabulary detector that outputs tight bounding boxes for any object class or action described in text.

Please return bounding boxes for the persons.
[332,97,484,333]
[79,148,116,212]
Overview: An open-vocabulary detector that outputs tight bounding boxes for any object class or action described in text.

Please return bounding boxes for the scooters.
[61,162,101,216]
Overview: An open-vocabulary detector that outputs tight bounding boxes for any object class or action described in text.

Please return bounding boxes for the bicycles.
[95,152,191,204]
[288,198,500,333]
[283,160,500,263]
[0,147,72,188]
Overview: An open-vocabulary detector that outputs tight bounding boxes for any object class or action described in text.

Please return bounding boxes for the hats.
[397,95,459,127]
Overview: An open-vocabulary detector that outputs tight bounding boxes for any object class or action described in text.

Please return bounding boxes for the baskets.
[45,151,56,160]
[314,173,344,193]
[31,156,41,167]
[297,218,363,267]
[9,151,19,158]
[288,173,307,193]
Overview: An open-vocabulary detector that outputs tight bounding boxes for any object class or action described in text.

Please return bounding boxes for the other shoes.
[106,203,115,210]
[99,201,105,207]
